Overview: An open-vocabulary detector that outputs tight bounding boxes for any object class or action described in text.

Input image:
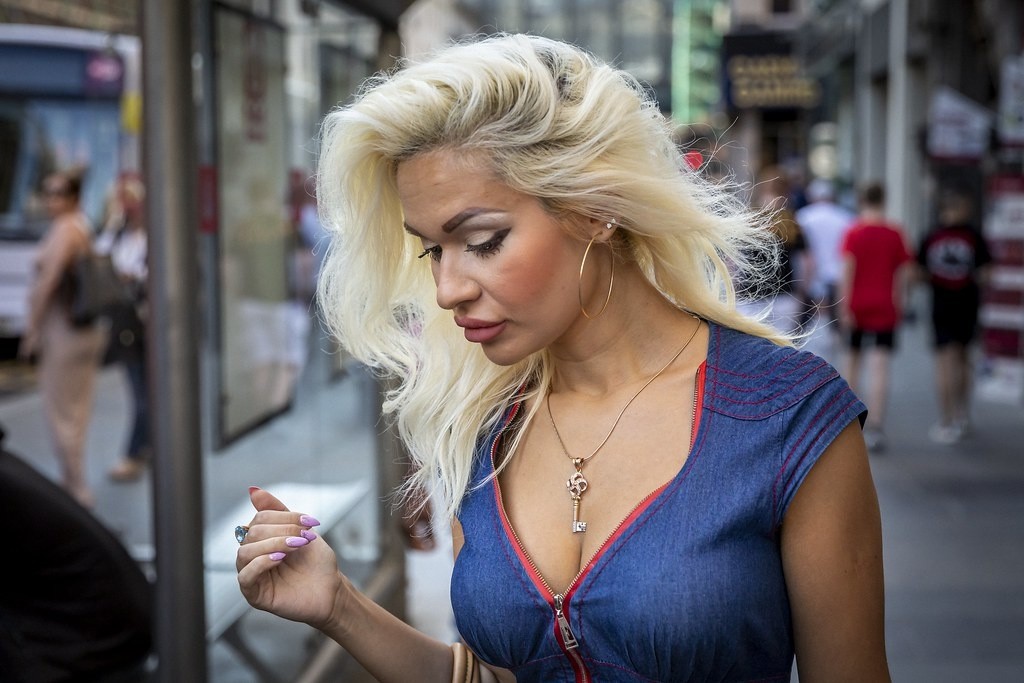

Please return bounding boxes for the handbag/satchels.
[69,256,127,321]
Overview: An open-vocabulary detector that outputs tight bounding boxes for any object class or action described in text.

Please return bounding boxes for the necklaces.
[546,308,701,533]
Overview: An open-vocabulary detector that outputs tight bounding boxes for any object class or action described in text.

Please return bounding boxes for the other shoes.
[110,461,143,482]
[927,422,965,444]
[862,424,885,450]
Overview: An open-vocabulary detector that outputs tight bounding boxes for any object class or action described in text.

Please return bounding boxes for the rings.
[234,526,250,545]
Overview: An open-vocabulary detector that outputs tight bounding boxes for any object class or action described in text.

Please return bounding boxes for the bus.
[0,22,142,340]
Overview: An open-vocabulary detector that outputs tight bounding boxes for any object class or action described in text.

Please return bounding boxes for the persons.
[22,164,148,506]
[683,130,988,457]
[0,428,160,682]
[237,33,892,682]
